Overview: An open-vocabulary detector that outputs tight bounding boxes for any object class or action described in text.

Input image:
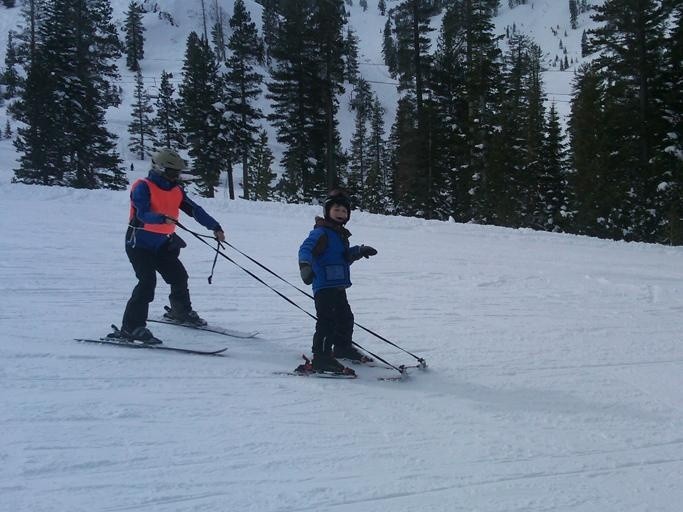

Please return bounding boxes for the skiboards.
[74,314,259,354]
[271,350,426,381]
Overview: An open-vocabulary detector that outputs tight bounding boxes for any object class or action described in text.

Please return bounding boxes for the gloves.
[360,243,377,259]
[299,263,317,285]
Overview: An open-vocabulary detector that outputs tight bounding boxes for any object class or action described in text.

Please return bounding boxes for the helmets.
[323,189,351,224]
[151,150,184,180]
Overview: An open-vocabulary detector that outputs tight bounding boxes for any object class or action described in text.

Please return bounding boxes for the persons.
[296,186,378,378]
[118,145,226,345]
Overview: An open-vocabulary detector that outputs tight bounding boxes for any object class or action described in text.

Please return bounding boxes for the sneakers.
[311,357,344,373]
[334,345,365,360]
[121,326,153,339]
[169,310,199,322]
